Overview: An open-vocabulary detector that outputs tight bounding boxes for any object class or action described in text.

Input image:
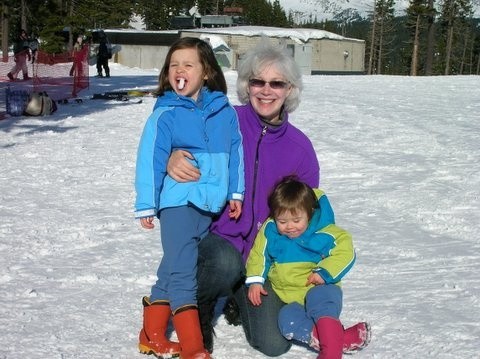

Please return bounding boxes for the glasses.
[249,78,290,89]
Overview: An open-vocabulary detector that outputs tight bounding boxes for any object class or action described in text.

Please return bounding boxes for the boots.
[139,296,182,359]
[317,318,345,358]
[171,305,210,359]
[309,322,371,354]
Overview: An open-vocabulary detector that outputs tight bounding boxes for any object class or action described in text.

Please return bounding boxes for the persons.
[69,28,92,77]
[198,37,319,359]
[244,174,372,359]
[7,30,32,81]
[94,29,112,79]
[132,37,246,359]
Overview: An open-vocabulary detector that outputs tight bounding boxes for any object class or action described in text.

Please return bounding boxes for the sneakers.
[224,299,241,325]
[198,321,214,353]
